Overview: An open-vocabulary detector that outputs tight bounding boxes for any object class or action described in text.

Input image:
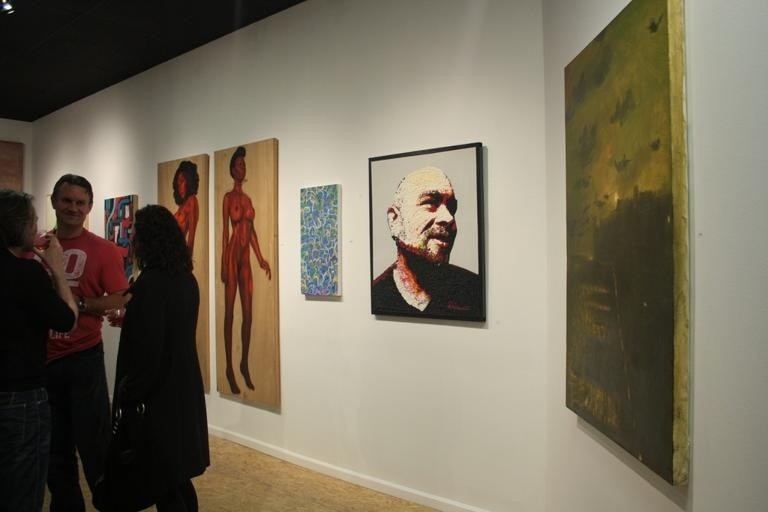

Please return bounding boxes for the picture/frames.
[368,142,487,323]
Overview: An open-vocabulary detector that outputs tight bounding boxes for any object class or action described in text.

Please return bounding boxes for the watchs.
[78,295,88,314]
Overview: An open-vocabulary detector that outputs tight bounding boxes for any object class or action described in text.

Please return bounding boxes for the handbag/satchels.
[90,396,165,512]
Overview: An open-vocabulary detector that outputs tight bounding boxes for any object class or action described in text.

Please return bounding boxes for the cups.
[106,303,126,326]
[34,230,53,252]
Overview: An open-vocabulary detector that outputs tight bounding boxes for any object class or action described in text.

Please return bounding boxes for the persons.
[170,161,198,269]
[0,186,80,510]
[22,172,134,510]
[220,146,271,393]
[99,205,211,510]
[374,166,481,313]
